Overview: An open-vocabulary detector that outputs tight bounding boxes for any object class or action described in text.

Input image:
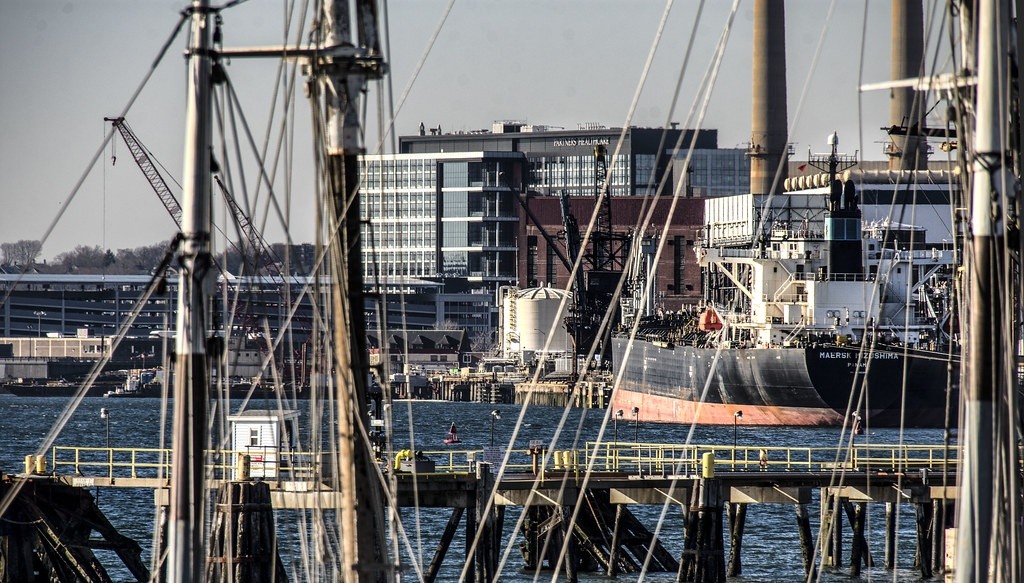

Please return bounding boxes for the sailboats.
[0,0,1024,583]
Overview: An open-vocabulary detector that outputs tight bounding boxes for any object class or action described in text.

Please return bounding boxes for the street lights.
[614,408,625,473]
[490,409,501,446]
[100,407,111,477]
[733,410,743,467]
[631,405,640,473]
[850,409,861,468]
